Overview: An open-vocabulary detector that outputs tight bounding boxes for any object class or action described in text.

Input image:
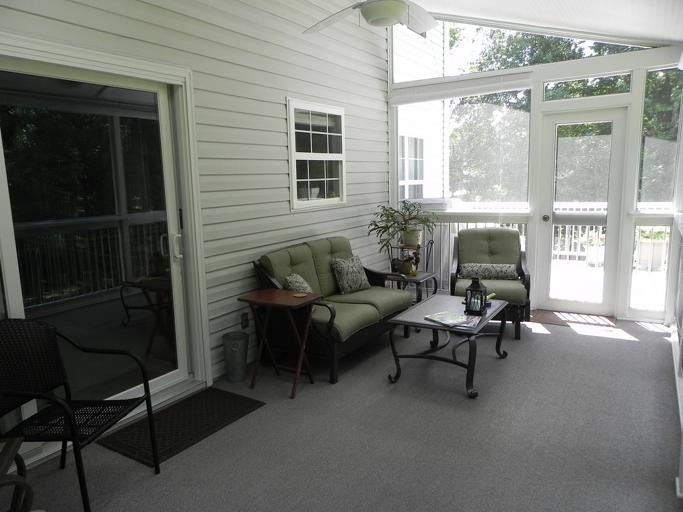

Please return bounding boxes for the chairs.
[0,317,160,512]
[449,228,530,321]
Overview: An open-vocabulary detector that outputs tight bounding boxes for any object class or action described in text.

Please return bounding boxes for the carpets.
[98,386,267,466]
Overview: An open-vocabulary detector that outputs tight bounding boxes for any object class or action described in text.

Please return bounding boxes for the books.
[422,309,473,329]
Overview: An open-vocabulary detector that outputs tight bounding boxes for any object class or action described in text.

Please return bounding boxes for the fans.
[304,0,437,38]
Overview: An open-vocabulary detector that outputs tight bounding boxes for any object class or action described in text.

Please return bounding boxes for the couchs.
[253,236,414,385]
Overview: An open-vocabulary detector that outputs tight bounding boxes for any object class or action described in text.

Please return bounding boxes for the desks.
[239,290,324,397]
[384,271,437,302]
[388,291,508,397]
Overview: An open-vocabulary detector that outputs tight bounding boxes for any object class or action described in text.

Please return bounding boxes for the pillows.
[457,262,519,280]
[282,273,314,297]
[325,255,372,296]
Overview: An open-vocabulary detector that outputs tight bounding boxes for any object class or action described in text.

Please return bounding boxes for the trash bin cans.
[222,332,250,383]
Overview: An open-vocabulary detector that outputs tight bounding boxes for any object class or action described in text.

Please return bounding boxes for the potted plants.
[369,199,439,247]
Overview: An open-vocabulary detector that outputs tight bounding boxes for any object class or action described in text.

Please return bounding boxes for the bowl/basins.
[297,187,319,198]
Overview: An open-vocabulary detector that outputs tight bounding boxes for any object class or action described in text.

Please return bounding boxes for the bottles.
[320,192,336,198]
[390,258,399,273]
